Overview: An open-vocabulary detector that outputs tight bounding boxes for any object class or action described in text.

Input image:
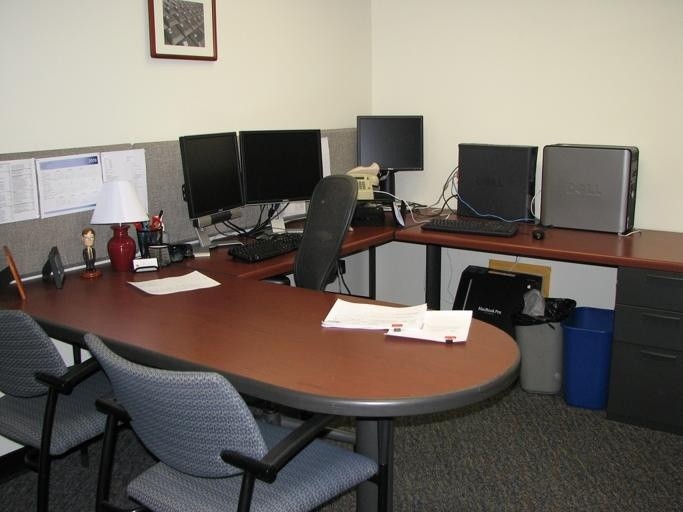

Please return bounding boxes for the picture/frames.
[3,246,26,301]
[42,246,65,288]
[148,0,218,61]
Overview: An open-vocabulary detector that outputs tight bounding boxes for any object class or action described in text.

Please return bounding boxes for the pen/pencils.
[137,212,163,254]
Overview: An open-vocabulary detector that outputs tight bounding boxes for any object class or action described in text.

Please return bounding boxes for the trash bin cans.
[564,306,614,412]
[515,295,571,396]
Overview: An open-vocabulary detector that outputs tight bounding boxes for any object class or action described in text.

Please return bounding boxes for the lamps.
[88,178,151,273]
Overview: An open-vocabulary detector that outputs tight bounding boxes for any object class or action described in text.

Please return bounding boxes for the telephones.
[350,174,379,200]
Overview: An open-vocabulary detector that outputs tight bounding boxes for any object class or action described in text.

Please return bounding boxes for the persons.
[81,227,102,280]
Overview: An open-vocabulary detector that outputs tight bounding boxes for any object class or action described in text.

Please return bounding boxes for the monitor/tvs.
[239,129,324,205]
[179,132,245,219]
[356,115,424,171]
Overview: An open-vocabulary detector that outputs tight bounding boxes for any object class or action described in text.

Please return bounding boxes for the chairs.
[0,310,112,511]
[83,333,395,512]
[293,176,359,291]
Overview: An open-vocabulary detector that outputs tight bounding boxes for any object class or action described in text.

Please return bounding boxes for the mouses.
[532,229,545,240]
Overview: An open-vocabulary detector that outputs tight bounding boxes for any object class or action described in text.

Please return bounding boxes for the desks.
[394,209,683,436]
[0,255,521,512]
[178,201,456,301]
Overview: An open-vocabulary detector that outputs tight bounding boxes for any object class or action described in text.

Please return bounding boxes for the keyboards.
[227,232,302,264]
[420,217,520,238]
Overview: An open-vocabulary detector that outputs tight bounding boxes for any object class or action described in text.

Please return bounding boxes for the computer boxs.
[539,143,640,234]
[457,142,539,222]
